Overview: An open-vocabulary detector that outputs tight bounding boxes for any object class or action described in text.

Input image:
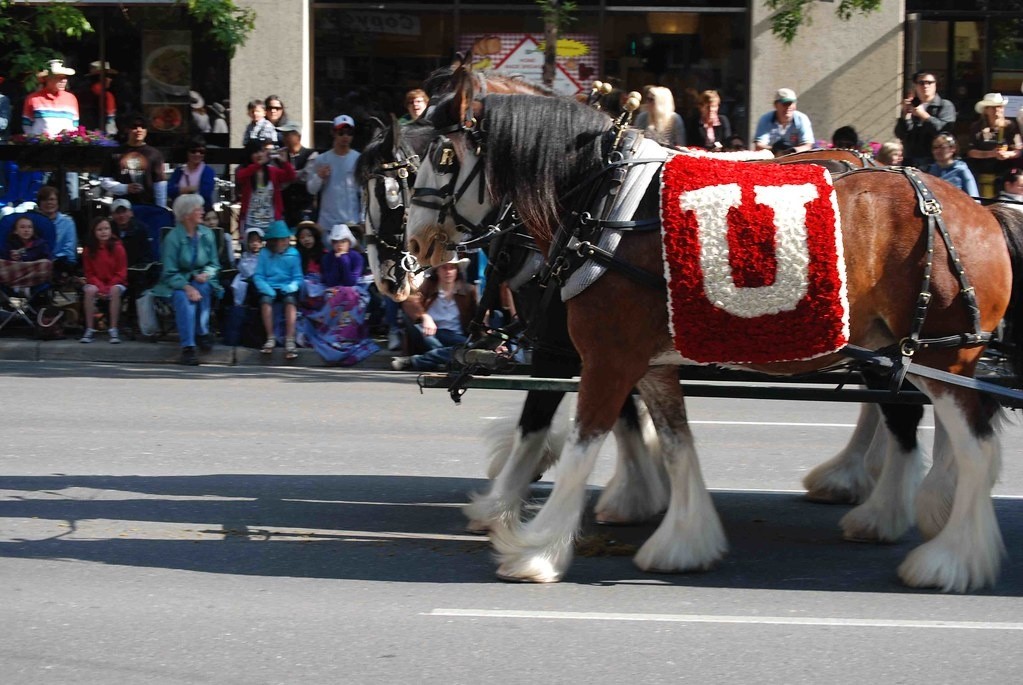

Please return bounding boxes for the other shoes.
[260,336,277,353]
[284,341,298,358]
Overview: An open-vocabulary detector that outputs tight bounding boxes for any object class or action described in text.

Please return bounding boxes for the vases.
[14,147,109,174]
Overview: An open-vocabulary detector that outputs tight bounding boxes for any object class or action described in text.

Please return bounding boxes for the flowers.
[2,125,120,152]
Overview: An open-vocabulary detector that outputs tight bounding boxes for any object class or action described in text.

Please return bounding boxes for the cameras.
[912,96,921,107]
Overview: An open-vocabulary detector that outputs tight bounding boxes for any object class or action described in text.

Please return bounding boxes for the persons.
[0,59,1023,370]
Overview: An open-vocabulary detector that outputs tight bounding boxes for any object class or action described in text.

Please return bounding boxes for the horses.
[352,50,1023,596]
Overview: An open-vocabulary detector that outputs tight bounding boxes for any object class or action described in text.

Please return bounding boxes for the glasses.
[334,128,354,136]
[265,105,283,110]
[914,79,936,85]
[127,122,148,130]
[187,147,207,153]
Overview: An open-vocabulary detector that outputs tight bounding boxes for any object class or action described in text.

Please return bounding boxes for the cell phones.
[268,151,281,158]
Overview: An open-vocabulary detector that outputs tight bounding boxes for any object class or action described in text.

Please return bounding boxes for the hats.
[35,58,77,76]
[975,90,1009,113]
[261,220,294,239]
[774,87,797,103]
[274,120,301,134]
[188,90,205,107]
[82,60,119,77]
[333,114,355,129]
[297,219,317,227]
[110,198,132,210]
[433,249,469,272]
[327,223,358,249]
[207,101,226,119]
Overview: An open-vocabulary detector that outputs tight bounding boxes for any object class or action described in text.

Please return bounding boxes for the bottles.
[510,339,525,364]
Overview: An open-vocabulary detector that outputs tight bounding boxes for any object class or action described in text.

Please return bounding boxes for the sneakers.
[107,328,122,343]
[181,349,198,366]
[79,328,95,342]
[198,336,213,350]
[389,355,408,370]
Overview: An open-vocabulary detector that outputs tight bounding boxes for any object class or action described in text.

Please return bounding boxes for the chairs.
[0,203,240,342]
